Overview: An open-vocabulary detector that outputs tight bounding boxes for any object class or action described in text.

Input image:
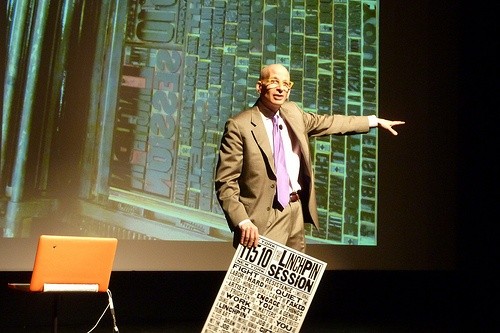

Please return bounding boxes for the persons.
[214,63,406,255]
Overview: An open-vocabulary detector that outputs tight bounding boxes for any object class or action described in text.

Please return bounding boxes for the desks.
[34,291,107,333]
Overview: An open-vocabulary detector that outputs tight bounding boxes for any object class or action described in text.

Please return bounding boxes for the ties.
[272,114,289,208]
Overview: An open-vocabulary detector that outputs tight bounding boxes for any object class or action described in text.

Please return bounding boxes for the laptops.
[8,234,117,293]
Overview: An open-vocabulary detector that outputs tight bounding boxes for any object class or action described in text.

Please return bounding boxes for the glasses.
[263,79,294,90]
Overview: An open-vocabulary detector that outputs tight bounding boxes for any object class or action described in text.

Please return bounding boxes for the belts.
[275,194,298,202]
